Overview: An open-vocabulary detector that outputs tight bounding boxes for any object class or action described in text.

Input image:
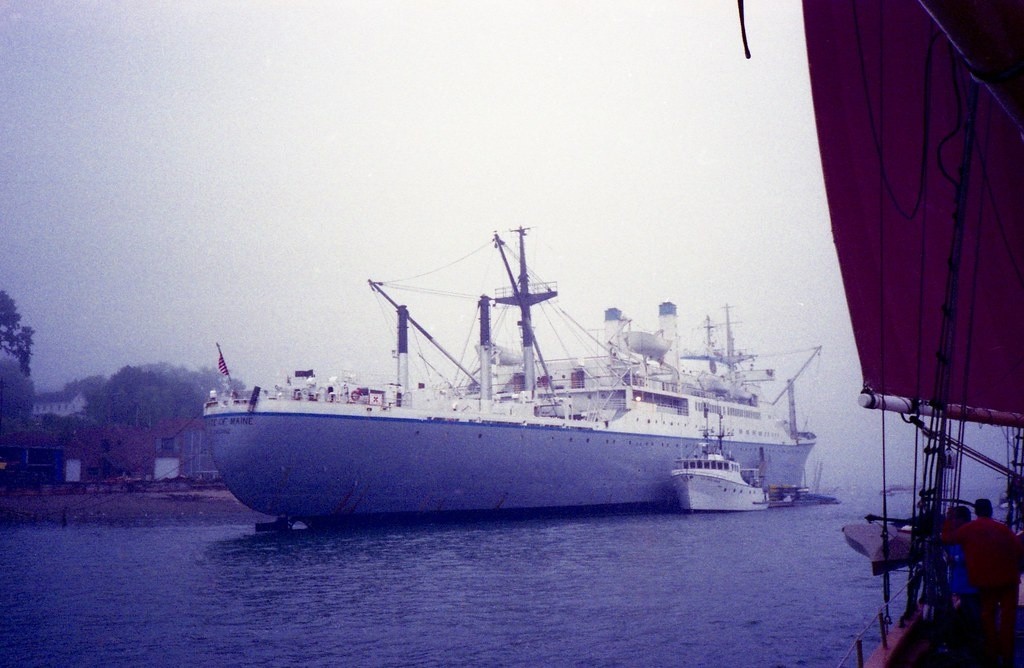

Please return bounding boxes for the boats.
[199,223,825,522]
[670,399,773,512]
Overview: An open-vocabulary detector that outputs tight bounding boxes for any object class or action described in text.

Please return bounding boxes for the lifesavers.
[350,390,360,401]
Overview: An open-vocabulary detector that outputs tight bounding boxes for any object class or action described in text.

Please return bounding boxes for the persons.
[779,488,801,501]
[940,497,1024,668]
[511,372,584,395]
[305,374,317,400]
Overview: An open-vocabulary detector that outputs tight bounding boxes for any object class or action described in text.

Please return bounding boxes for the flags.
[219,351,229,376]
